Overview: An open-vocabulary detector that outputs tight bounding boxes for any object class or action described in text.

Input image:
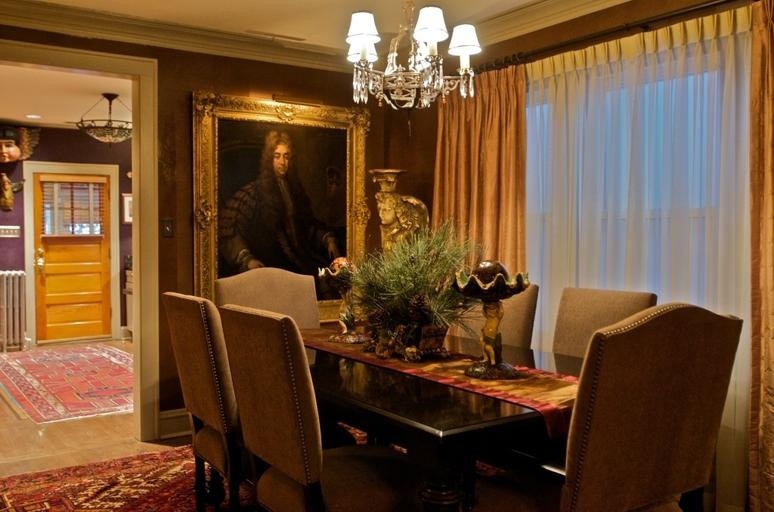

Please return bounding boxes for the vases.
[367,166,431,258]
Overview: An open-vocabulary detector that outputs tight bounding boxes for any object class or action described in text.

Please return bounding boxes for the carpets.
[0,421,566,511]
[0,341,134,426]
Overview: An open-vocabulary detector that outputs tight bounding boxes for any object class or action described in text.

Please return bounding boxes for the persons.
[218,130,341,297]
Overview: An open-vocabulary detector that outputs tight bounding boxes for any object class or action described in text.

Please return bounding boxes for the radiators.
[0,271,27,354]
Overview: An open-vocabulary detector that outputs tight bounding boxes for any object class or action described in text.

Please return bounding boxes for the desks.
[288,317,599,511]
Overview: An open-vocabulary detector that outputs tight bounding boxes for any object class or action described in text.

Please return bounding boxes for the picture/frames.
[122,191,134,224]
[189,89,371,325]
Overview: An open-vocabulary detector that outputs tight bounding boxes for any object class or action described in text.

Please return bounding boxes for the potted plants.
[350,219,485,366]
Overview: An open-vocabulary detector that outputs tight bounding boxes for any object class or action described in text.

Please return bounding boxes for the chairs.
[160,290,358,511]
[552,285,660,358]
[445,281,542,348]
[219,301,424,511]
[210,265,326,371]
[463,300,745,510]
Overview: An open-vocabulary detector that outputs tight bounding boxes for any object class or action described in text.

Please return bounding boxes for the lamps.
[339,0,486,112]
[66,91,134,146]
